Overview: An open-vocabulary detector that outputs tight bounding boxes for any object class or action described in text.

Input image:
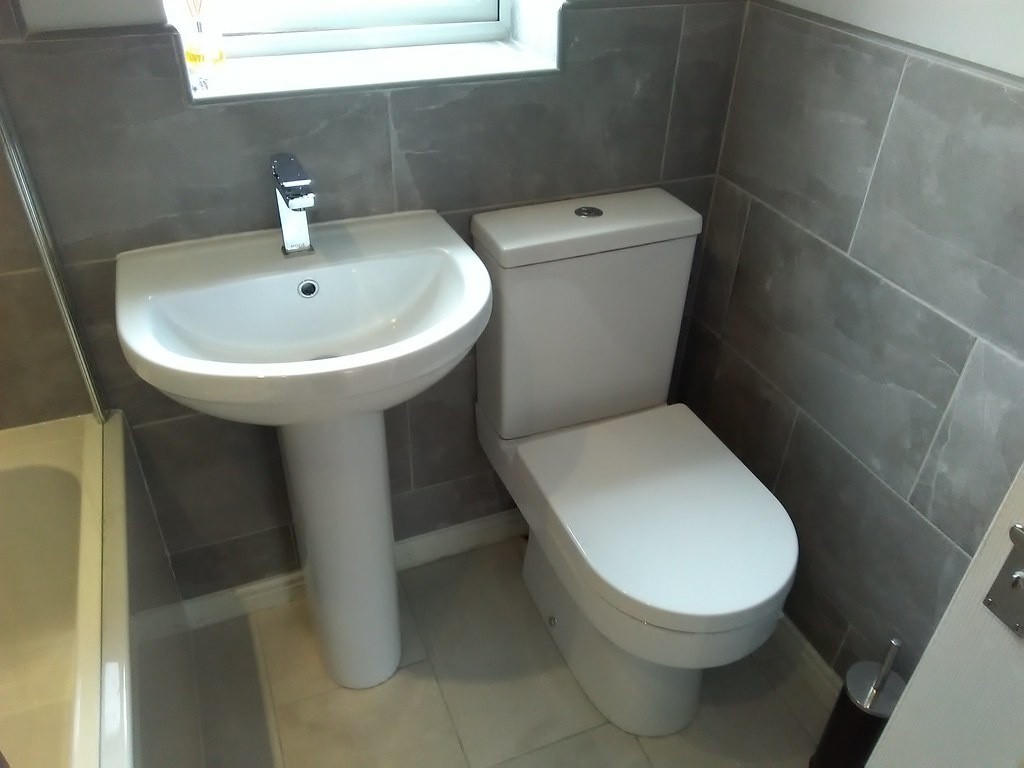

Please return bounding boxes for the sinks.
[116,212,491,422]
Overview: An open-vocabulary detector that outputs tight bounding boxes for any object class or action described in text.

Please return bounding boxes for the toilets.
[474,186,797,740]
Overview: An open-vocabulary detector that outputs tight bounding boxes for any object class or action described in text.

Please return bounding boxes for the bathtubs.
[0,412,194,767]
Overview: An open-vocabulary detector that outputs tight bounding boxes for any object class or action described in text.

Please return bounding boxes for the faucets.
[270,154,318,257]
